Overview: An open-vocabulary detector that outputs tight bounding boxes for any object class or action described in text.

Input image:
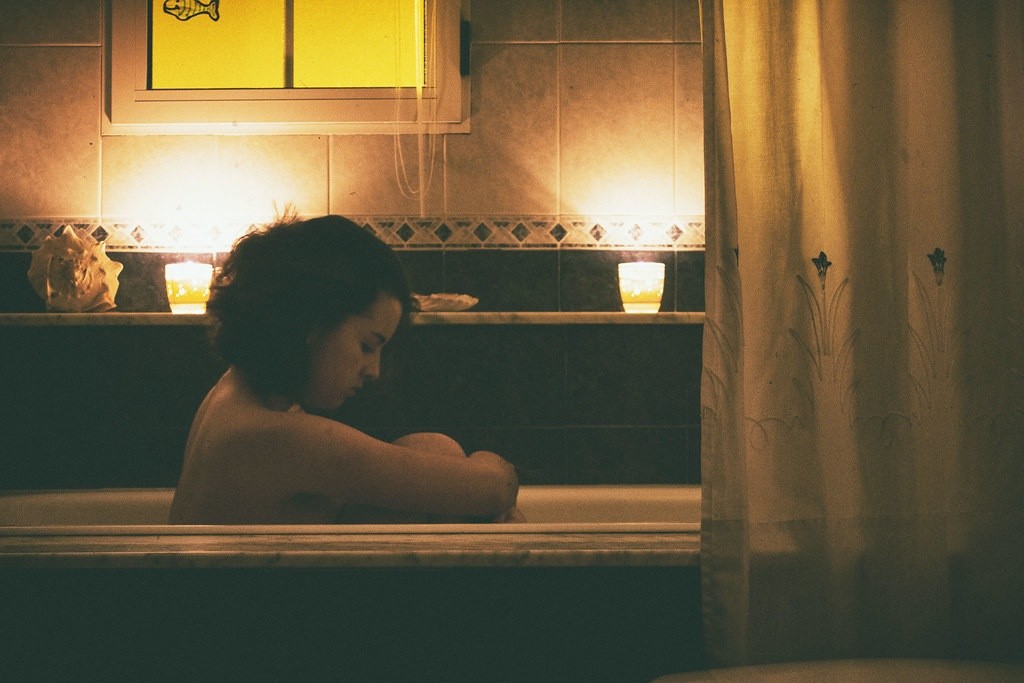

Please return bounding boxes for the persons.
[166,210,529,526]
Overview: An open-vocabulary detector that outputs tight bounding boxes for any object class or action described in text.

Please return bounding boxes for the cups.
[163,262,209,314]
[618,262,668,314]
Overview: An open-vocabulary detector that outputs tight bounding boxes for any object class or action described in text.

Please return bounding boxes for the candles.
[618,262,665,314]
[166,262,212,315]
